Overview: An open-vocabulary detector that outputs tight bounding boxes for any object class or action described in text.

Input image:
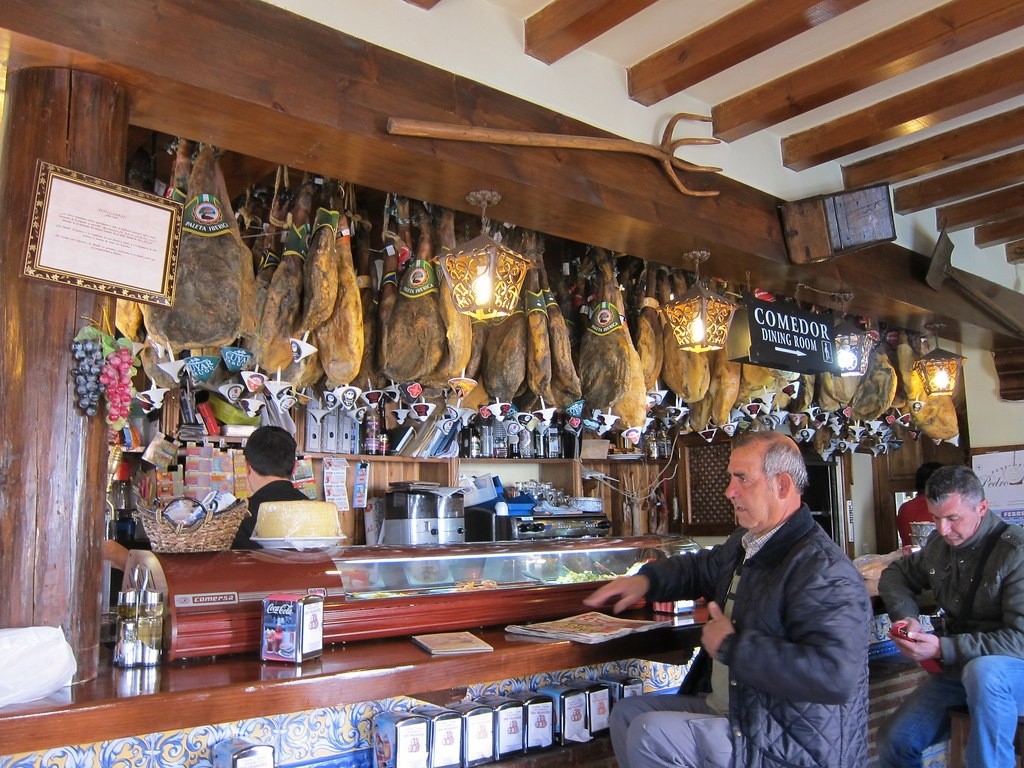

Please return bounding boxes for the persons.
[580,431,875,768]
[879,467,1023,768]
[896,463,949,545]
[229,426,312,552]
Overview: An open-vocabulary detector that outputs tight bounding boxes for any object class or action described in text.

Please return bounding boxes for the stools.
[949,704,1024,768]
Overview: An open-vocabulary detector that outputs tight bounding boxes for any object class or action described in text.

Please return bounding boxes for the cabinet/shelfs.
[102,446,677,614]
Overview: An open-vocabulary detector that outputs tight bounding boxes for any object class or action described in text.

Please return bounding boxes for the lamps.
[911,322,969,397]
[665,251,740,354]
[831,293,878,378]
[439,189,534,319]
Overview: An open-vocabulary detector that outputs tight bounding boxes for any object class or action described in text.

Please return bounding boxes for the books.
[412,632,493,654]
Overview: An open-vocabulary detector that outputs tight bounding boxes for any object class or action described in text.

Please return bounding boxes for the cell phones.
[889,623,915,642]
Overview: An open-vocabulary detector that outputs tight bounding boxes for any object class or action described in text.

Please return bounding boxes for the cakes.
[256,500,339,537]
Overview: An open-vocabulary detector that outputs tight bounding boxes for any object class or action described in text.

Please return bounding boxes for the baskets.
[131,498,252,553]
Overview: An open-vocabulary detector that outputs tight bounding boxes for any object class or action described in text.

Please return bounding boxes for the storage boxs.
[306,398,352,453]
[208,390,261,426]
[463,474,538,515]
[372,673,644,768]
[260,594,323,663]
[654,600,695,615]
[212,736,275,768]
